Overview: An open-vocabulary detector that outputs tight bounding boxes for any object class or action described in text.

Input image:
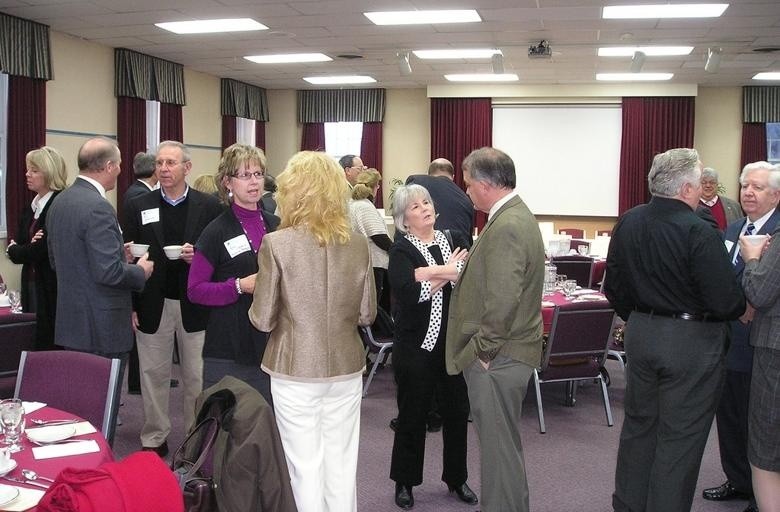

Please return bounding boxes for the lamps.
[630,47,647,78]
[702,46,726,76]
[490,51,506,75]
[395,51,414,80]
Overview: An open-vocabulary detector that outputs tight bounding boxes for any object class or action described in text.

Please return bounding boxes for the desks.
[542,238,615,258]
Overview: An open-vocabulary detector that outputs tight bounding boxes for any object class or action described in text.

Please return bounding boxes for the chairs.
[595,228,615,237]
[549,254,594,289]
[526,294,619,434]
[605,314,633,385]
[174,378,299,512]
[566,238,593,257]
[11,347,123,446]
[357,306,394,396]
[0,309,43,399]
[33,447,186,512]
[556,226,587,240]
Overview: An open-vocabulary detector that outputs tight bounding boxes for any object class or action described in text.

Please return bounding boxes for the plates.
[0,485,20,508]
[29,426,75,439]
[541,289,602,309]
[0,457,19,478]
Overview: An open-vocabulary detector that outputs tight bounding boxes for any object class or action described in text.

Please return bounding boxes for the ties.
[734,224,755,267]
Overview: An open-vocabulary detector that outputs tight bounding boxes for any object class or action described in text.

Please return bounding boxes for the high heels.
[396,482,414,508]
[449,482,478,504]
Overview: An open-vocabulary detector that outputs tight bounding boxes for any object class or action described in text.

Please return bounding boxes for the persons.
[388,182,478,510]
[351,168,397,376]
[194,170,279,215]
[603,147,755,512]
[119,150,158,238]
[702,160,780,502]
[247,149,378,512]
[737,222,780,511]
[338,154,369,190]
[188,142,282,406]
[118,138,223,470]
[5,147,68,351]
[394,156,474,255]
[699,166,743,235]
[46,138,157,447]
[444,146,543,512]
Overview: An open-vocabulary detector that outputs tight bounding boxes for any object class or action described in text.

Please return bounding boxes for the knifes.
[1,473,50,489]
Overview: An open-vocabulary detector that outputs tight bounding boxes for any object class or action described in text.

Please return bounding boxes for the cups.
[160,245,187,261]
[745,235,769,246]
[577,245,587,255]
[128,242,147,257]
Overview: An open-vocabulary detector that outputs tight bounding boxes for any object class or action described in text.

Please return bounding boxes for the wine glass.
[543,264,578,302]
[0,398,27,453]
[0,283,23,312]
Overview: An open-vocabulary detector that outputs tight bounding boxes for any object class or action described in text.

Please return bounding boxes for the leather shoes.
[702,480,749,500]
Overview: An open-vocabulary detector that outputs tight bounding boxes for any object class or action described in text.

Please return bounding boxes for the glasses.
[231,171,264,180]
[155,160,189,168]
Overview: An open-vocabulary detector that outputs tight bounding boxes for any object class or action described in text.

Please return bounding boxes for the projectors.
[528,40,552,60]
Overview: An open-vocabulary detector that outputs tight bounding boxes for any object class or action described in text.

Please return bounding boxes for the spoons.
[21,467,54,484]
[31,418,79,424]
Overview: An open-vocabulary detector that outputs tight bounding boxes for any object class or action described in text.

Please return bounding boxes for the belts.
[638,307,718,322]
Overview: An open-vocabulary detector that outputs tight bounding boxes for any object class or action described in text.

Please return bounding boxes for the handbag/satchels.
[166,417,220,512]
